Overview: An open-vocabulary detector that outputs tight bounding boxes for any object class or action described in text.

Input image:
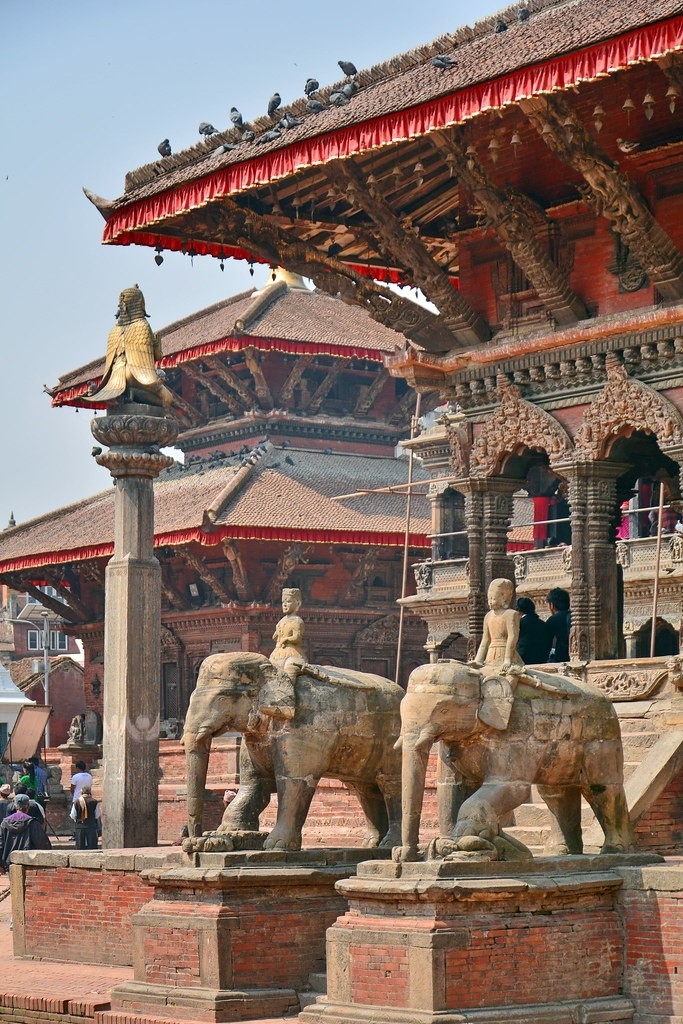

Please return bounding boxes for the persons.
[68,760,93,840]
[545,490,683,551]
[258,587,306,719]
[68,785,102,850]
[544,588,571,664]
[466,577,525,693]
[0,793,53,876]
[0,757,50,840]
[515,597,547,665]
